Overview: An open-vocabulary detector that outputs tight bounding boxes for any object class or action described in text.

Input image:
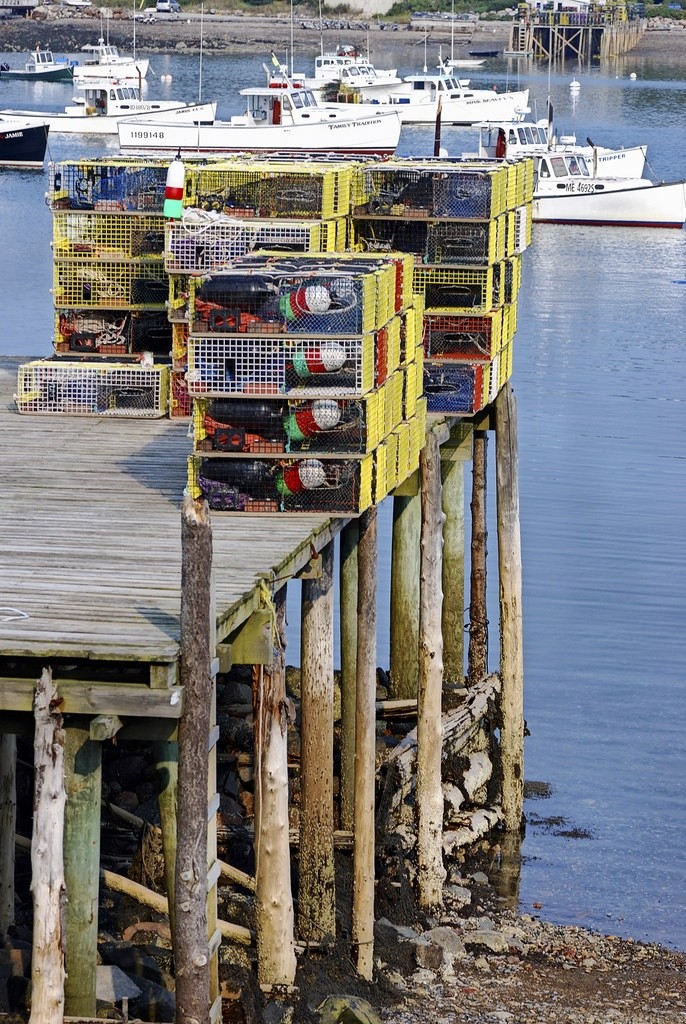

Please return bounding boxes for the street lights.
[338,11,346,31]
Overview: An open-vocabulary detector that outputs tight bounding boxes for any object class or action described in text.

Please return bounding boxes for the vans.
[156,0,181,12]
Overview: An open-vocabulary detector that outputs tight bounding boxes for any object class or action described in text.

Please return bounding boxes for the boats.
[398,95,686,231]
[469,50,500,56]
[264,0,402,89]
[74,16,150,77]
[117,108,404,163]
[0,47,75,80]
[230,64,530,126]
[0,125,50,169]
[0,66,246,134]
[447,59,487,65]
